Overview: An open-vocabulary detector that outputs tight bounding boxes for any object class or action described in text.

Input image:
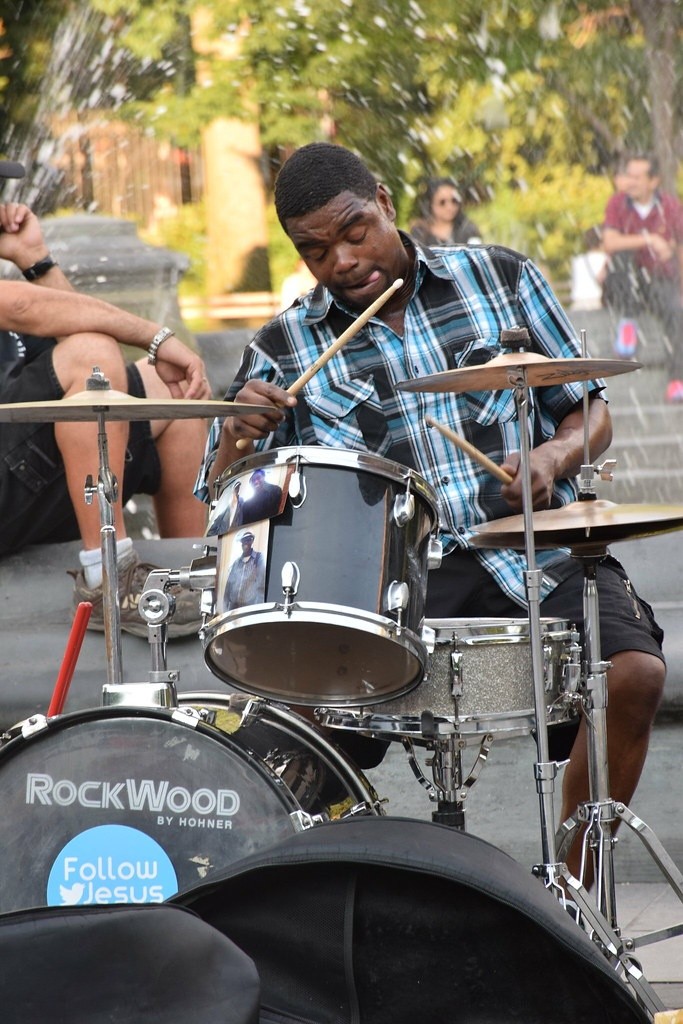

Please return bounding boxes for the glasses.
[441,197,457,206]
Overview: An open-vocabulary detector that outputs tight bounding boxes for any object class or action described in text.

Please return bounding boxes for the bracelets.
[21,252,57,282]
[146,328,176,366]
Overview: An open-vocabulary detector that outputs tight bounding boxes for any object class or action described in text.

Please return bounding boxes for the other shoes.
[614,317,637,359]
[665,379,683,403]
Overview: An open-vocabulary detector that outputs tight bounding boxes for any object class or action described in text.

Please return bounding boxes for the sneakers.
[65,551,203,639]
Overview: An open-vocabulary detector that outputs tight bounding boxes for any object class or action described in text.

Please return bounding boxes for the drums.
[315,617,586,734]
[0,689,382,917]
[200,445,441,706]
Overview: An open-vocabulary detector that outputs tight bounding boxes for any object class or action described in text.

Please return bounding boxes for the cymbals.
[467,502,683,551]
[0,390,277,423]
[393,351,645,394]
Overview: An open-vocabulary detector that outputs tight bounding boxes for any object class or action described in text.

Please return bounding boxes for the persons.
[0,160,213,641]
[599,154,683,404]
[206,469,283,612]
[409,178,484,247]
[193,143,668,928]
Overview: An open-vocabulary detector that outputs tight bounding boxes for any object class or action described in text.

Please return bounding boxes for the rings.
[203,377,207,384]
